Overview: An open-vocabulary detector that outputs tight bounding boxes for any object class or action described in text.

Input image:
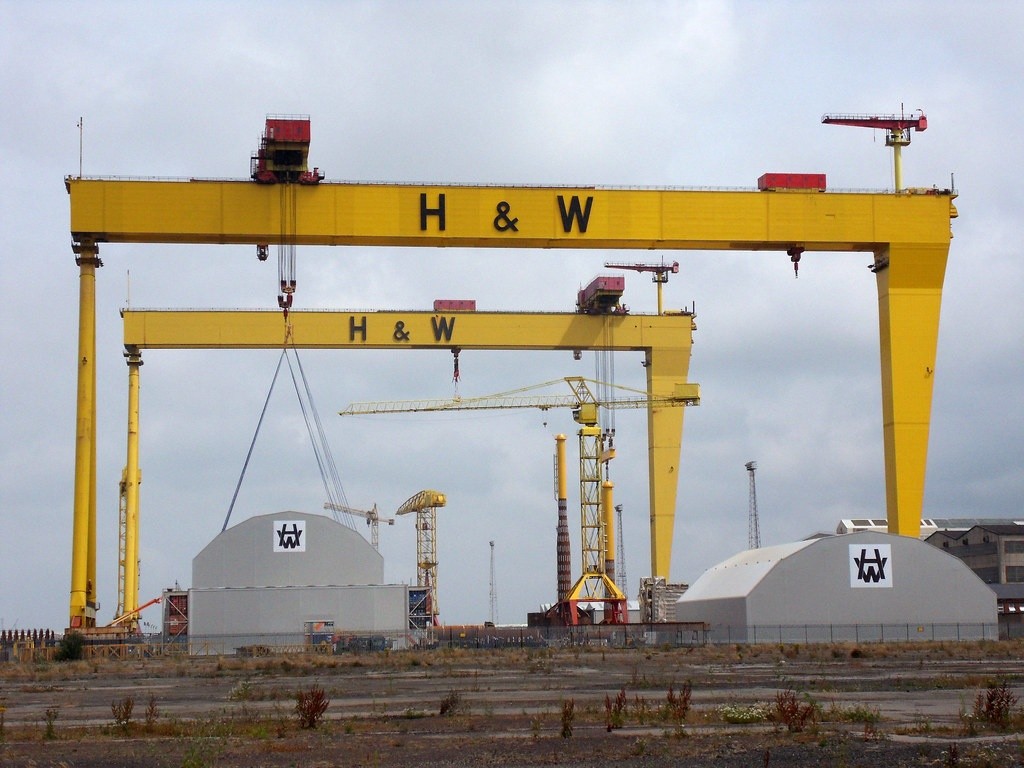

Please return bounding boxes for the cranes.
[323,502,395,549]
[333,373,700,653]
